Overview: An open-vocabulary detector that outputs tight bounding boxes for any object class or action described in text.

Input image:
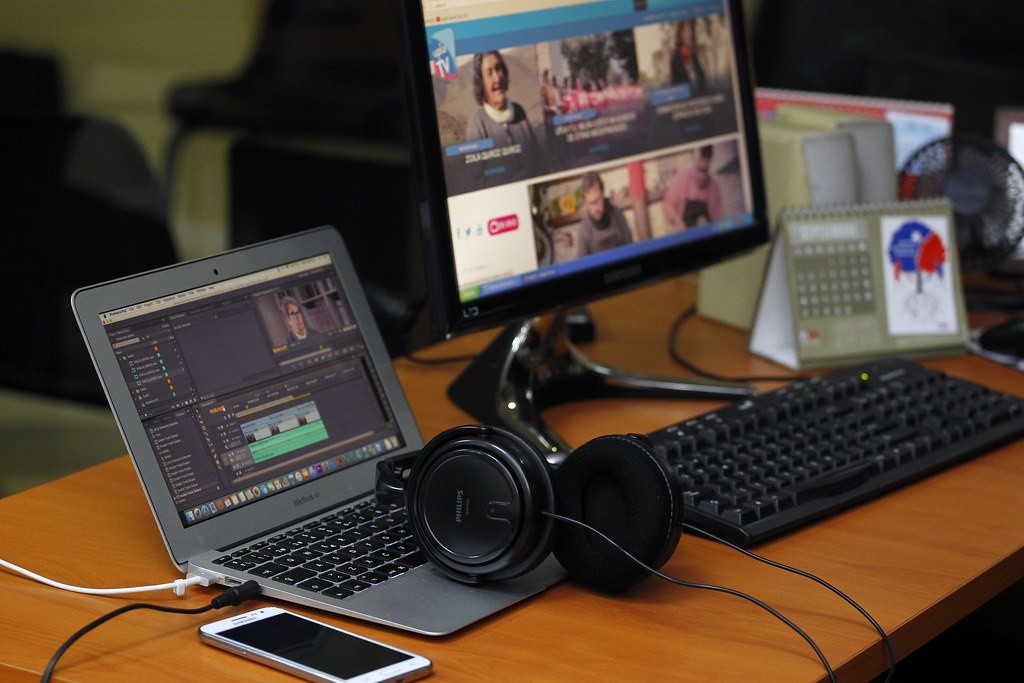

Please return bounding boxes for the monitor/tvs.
[392,0,771,339]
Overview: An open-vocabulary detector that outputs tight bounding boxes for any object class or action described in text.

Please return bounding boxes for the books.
[695,117,861,339]
[773,98,902,207]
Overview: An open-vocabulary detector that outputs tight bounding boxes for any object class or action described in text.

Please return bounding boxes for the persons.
[575,171,633,260]
[544,64,642,123]
[659,142,727,235]
[279,295,323,345]
[465,49,541,162]
[668,17,707,103]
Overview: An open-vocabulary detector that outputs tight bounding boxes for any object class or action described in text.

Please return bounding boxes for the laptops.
[73,222,569,637]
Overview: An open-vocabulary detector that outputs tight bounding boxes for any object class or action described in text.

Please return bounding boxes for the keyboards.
[644,357,1023,550]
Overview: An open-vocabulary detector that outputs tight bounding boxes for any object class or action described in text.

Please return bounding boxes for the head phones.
[373,424,685,599]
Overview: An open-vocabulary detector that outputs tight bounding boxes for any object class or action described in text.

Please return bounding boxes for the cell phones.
[197,606,434,683]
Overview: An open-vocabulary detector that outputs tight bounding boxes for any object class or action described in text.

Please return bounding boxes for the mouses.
[979,315,1024,358]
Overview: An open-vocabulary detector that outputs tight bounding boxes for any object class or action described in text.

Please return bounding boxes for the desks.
[1,259,1023,683]
